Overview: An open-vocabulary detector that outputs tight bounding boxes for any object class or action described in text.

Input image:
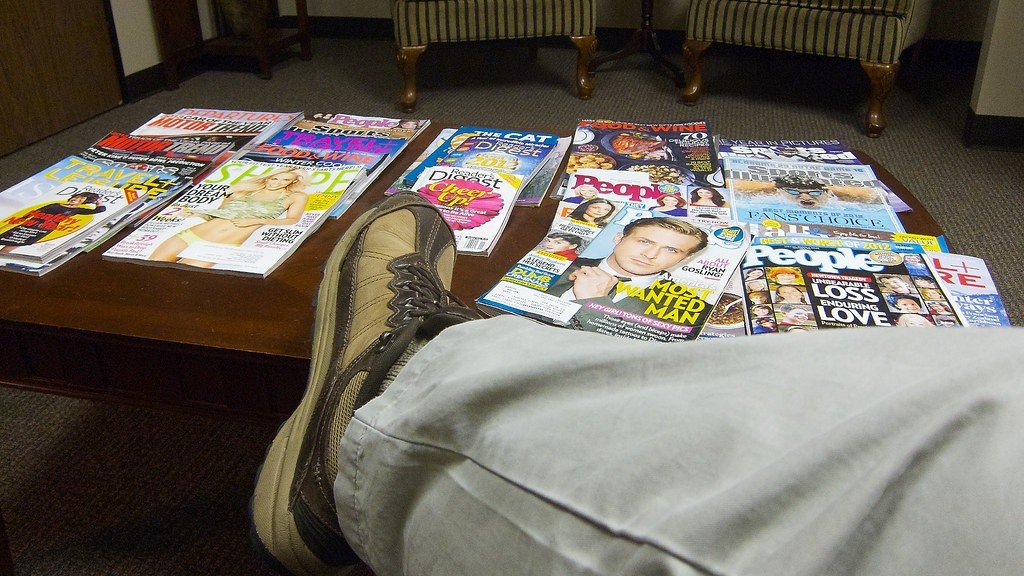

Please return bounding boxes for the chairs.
[388,0,598,114]
[678,0,932,139]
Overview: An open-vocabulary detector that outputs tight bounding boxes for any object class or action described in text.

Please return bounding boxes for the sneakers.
[249,191,483,576]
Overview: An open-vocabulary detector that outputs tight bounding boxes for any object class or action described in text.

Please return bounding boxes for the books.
[288,110,432,220]
[757,217,949,254]
[718,138,906,233]
[102,129,408,279]
[925,252,1011,327]
[172,109,305,163]
[734,242,970,335]
[0,131,234,277]
[474,120,755,341]
[129,113,275,227]
[384,126,572,257]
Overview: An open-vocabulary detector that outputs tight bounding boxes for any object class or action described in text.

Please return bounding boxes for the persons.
[148,166,313,268]
[545,216,713,337]
[690,187,728,206]
[878,254,960,327]
[567,198,615,226]
[726,173,882,209]
[746,267,811,332]
[563,185,599,204]
[649,193,687,216]
[248,189,1024,576]
[546,232,582,260]
[0,192,99,245]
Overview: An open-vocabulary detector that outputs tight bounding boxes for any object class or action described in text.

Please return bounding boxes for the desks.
[0,104,1014,428]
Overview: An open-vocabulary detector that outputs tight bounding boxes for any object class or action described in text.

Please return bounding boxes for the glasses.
[781,188,825,196]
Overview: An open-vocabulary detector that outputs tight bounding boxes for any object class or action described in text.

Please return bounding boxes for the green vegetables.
[635,132,650,139]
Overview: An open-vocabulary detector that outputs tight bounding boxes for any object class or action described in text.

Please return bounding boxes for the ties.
[607,276,631,300]
